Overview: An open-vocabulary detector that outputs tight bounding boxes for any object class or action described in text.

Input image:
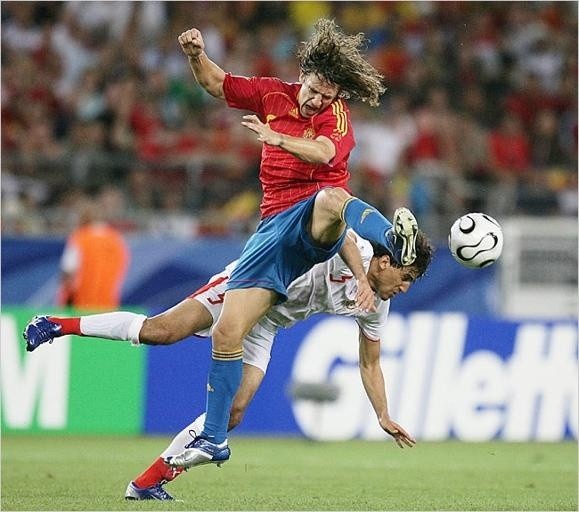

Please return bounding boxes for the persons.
[51,202,131,310]
[2,3,577,239]
[22,226,433,503]
[166,25,419,470]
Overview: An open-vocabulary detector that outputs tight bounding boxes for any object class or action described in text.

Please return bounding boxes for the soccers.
[448,213,504,268]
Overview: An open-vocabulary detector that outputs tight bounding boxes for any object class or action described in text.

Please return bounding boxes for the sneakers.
[163,436,231,467]
[385,208,419,268]
[23,315,64,352]
[125,481,184,504]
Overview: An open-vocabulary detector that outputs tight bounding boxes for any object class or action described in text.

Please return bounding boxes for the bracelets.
[279,134,285,147]
